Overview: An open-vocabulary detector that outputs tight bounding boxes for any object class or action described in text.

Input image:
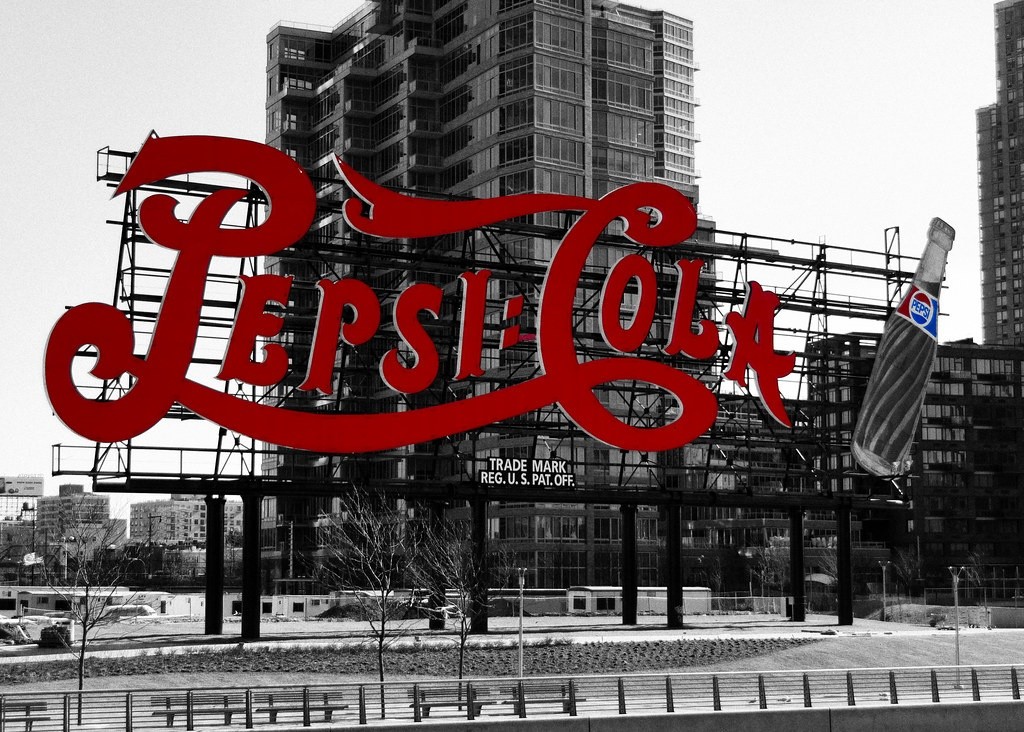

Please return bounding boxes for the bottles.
[851,218,956,481]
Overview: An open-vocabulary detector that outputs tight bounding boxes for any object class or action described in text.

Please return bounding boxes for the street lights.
[517,568,527,678]
[61,536,74,579]
[948,567,965,690]
[878,560,891,621]
